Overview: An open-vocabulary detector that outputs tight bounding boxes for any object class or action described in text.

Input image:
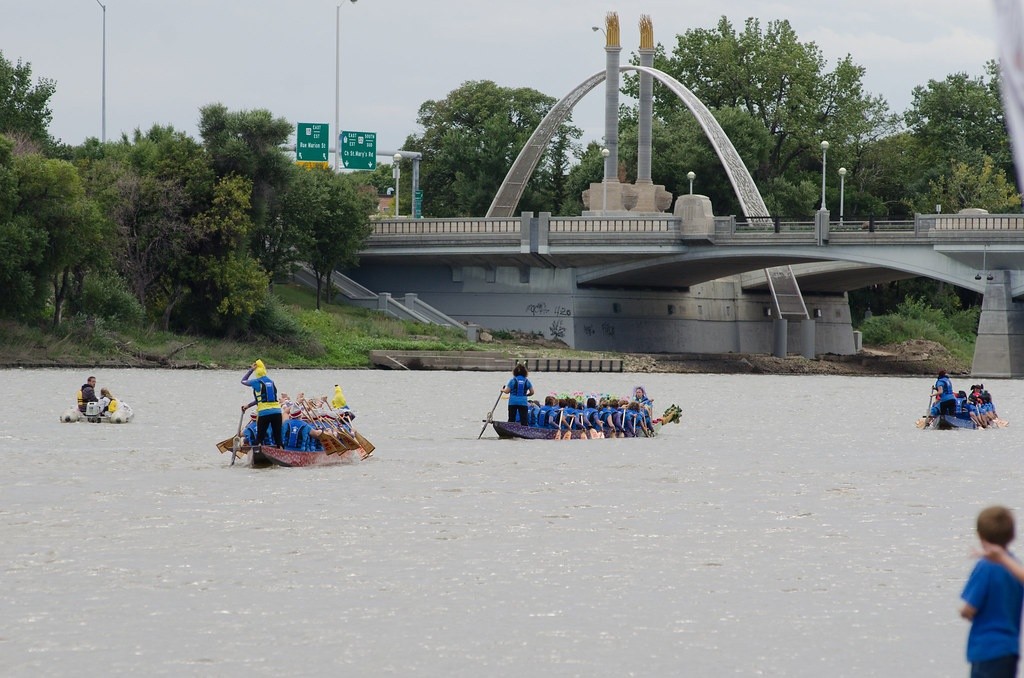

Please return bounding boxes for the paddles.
[298,391,375,461]
[916,416,934,430]
[218,393,294,459]
[966,403,1010,430]
[554,407,652,440]
[230,406,244,466]
[477,384,505,440]
[924,384,934,427]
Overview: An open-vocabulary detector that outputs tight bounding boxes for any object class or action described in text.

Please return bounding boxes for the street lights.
[687,170,696,195]
[335,0,357,171]
[819,140,830,211]
[600,148,611,217]
[838,166,847,227]
[392,153,402,217]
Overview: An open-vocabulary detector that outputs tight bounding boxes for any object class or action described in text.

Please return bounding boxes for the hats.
[289,402,302,418]
[250,409,257,420]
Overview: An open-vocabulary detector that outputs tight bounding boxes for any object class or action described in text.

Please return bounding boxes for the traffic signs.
[295,123,329,163]
[339,130,377,171]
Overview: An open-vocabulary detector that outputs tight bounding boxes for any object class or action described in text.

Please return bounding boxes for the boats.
[495,417,655,441]
[915,404,1007,431]
[240,423,375,468]
[59,399,134,426]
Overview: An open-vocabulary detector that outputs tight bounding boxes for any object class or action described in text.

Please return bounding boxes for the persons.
[500,364,655,433]
[77,377,117,417]
[959,506,1024,678]
[240,358,355,452]
[927,369,997,427]
